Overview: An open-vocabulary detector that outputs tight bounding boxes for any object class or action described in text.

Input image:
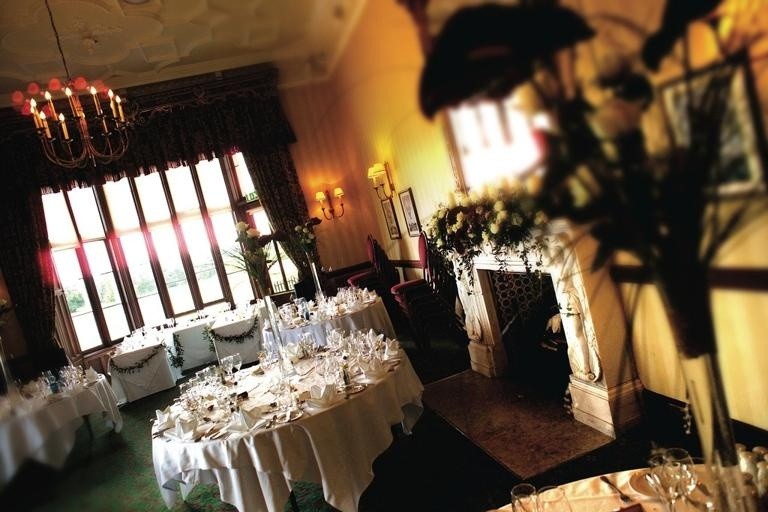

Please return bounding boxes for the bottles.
[47,369,59,394]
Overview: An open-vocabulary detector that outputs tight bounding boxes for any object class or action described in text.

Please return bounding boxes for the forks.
[599,475,633,504]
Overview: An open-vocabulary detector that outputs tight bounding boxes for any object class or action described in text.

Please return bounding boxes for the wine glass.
[176,353,243,420]
[335,286,362,312]
[679,457,714,511]
[60,361,85,398]
[661,448,696,512]
[329,326,376,378]
[277,296,309,329]
[642,452,682,512]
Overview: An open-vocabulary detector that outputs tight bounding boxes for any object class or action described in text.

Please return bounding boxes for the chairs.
[345,232,464,356]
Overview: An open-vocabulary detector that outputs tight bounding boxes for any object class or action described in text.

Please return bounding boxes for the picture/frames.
[398,187,422,238]
[656,44,768,202]
[380,198,403,240]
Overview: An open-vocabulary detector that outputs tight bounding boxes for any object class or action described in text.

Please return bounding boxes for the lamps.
[28,1,130,170]
[314,187,348,222]
[365,162,396,200]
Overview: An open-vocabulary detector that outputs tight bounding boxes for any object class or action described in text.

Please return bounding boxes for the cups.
[510,483,536,512]
[534,486,572,512]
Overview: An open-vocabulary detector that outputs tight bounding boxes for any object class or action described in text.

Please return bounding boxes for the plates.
[628,467,681,501]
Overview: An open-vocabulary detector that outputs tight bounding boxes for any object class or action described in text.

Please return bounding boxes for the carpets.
[414,369,616,482]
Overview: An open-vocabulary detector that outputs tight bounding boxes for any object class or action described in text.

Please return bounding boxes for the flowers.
[398,0,768,357]
[292,225,317,261]
[423,175,554,296]
[217,219,292,276]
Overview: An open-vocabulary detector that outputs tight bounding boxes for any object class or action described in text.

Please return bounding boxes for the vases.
[255,275,273,300]
[674,350,742,466]
[305,251,327,303]
